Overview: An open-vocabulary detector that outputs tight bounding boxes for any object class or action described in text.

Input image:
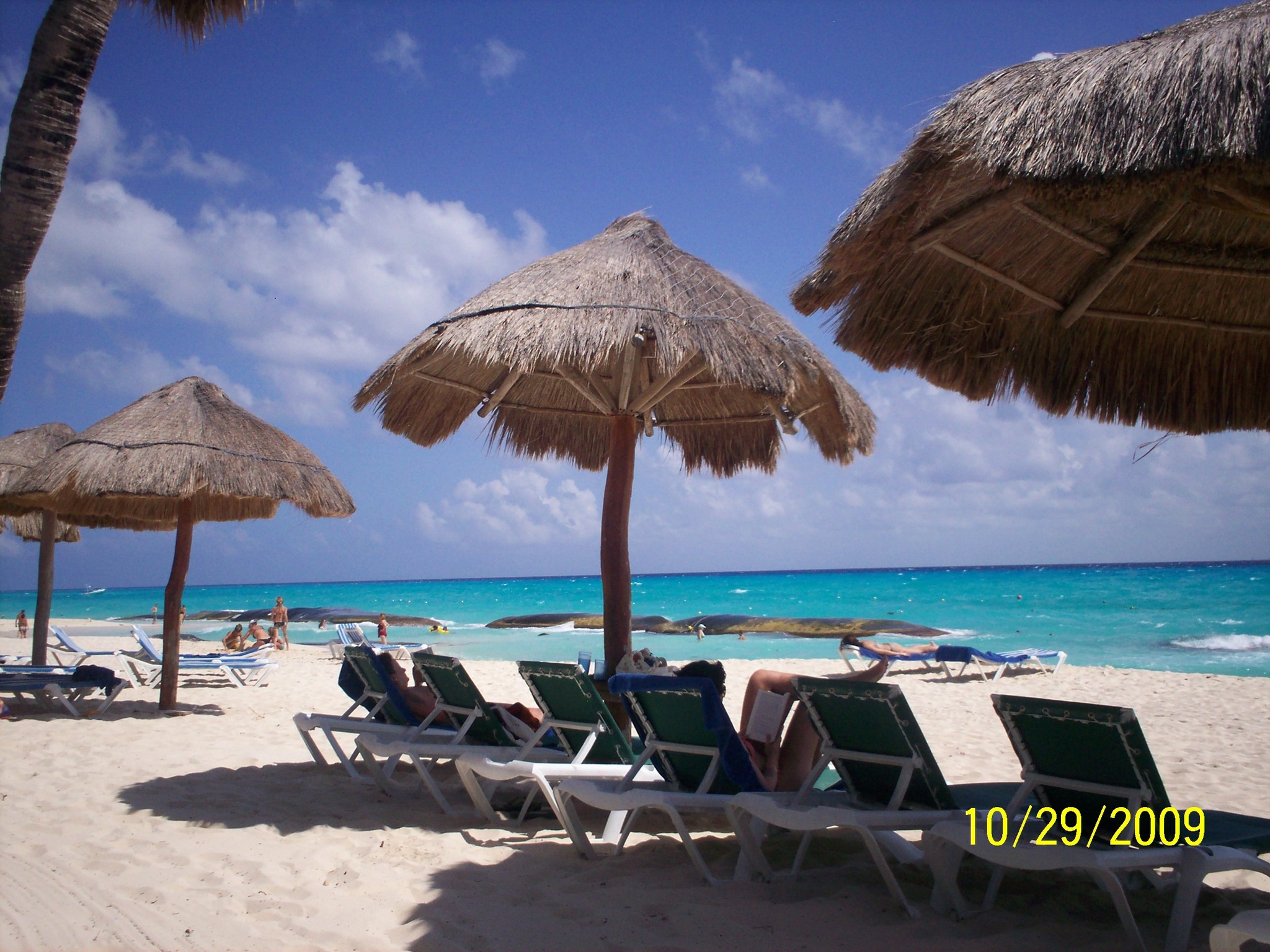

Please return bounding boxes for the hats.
[249,619,256,628]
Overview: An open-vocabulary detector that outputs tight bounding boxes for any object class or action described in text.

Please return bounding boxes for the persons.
[15,609,26,638]
[150,603,157,624]
[430,625,448,633]
[18,614,29,639]
[841,634,951,657]
[673,650,896,797]
[318,619,328,630]
[222,623,245,652]
[269,597,290,650]
[377,613,390,653]
[375,650,547,735]
[179,605,186,626]
[267,627,285,650]
[240,619,272,652]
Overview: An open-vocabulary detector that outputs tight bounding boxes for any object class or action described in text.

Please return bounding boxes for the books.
[745,687,792,745]
[407,643,436,682]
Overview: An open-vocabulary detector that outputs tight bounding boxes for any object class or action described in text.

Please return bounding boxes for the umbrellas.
[0,421,107,675]
[358,204,881,752]
[789,0,1270,443]
[4,377,357,709]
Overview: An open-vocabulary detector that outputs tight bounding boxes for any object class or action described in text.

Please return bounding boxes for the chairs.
[723,676,1048,923]
[114,629,284,690]
[837,641,944,674]
[131,624,274,674]
[328,623,371,660]
[453,658,665,844]
[293,645,466,786]
[336,627,431,660]
[921,689,1270,952]
[551,672,924,887]
[352,651,569,820]
[935,644,1070,682]
[0,654,130,717]
[46,623,114,668]
